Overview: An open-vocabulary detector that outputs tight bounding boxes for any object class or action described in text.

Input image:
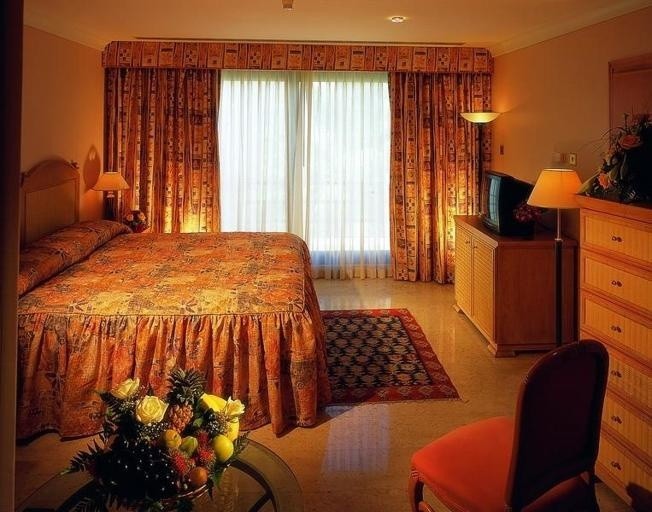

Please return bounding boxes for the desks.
[14,434,305,512]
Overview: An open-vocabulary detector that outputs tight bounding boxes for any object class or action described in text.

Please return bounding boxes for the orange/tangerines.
[191,467,209,486]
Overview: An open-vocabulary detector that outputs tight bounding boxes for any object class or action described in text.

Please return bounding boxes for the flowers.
[61,368,247,495]
[122,211,151,233]
[577,112,652,205]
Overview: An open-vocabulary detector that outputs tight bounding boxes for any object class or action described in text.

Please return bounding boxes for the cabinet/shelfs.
[575,193,652,510]
[452,214,578,358]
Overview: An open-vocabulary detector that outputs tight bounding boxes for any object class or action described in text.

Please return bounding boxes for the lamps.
[91,171,130,221]
[526,167,586,242]
[460,111,508,124]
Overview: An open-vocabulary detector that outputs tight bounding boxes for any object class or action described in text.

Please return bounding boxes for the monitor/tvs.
[482,170,535,237]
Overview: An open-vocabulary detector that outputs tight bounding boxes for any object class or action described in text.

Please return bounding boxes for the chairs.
[407,338,609,512]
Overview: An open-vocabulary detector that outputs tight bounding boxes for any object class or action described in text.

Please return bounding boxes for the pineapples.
[163,366,209,432]
[203,411,228,439]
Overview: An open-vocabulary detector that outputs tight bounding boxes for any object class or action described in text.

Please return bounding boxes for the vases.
[123,482,212,511]
[615,158,652,203]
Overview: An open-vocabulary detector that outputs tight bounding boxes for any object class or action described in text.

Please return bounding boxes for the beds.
[16,157,331,441]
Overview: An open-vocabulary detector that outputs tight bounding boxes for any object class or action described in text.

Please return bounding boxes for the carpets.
[320,306,462,405]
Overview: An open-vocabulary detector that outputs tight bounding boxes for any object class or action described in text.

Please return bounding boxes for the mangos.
[212,435,235,463]
